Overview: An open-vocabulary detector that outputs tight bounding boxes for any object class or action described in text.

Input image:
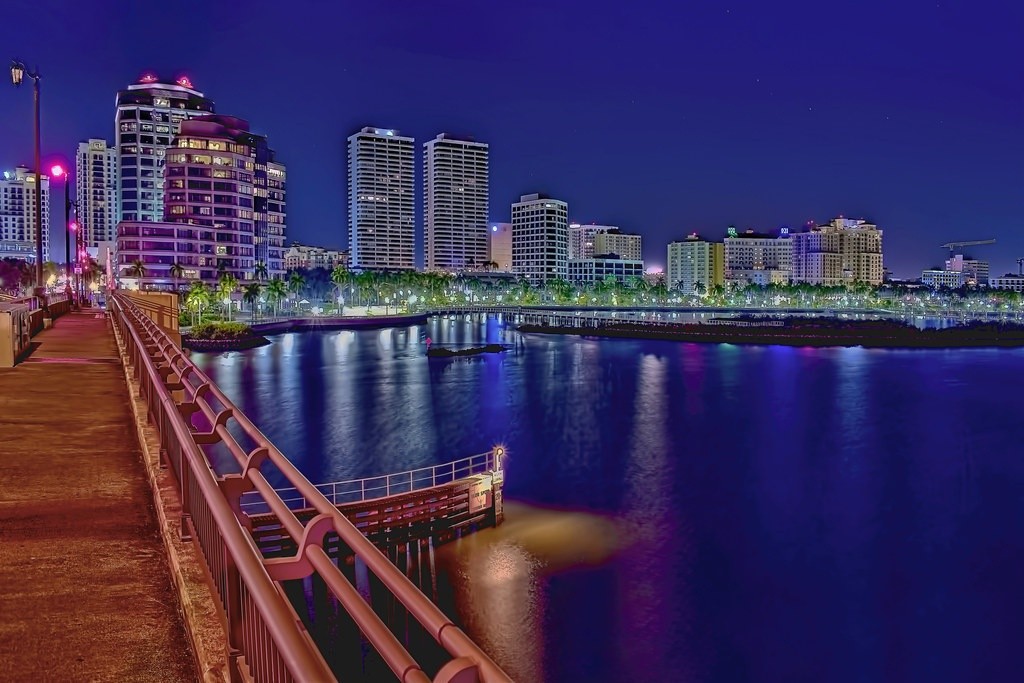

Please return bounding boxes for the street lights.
[223,299,229,320]
[52,166,71,303]
[69,200,87,307]
[9,57,45,296]
[258,297,265,318]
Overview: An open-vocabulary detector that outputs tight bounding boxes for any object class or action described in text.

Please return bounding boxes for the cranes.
[939,240,997,258]
